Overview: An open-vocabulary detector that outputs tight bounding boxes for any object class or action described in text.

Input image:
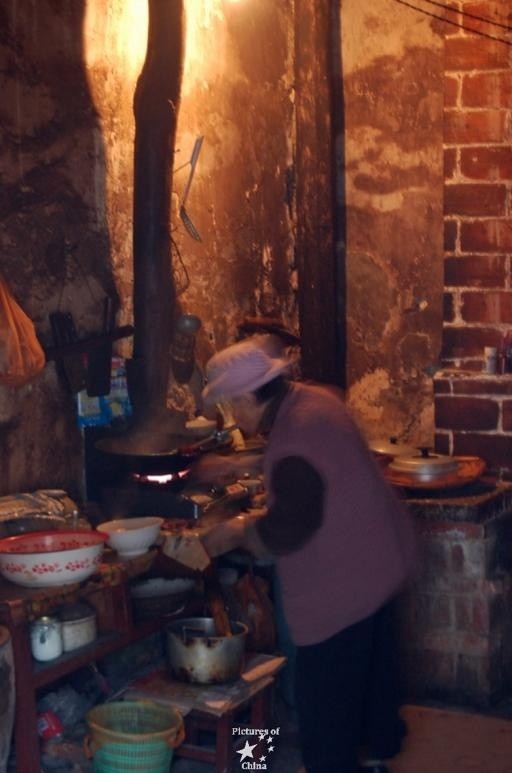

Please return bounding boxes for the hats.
[198,340,293,407]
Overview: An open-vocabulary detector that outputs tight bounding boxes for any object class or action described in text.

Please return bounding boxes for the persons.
[186,338,429,772]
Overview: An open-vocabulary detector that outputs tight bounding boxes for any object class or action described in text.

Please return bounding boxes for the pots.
[164,615,249,687]
[93,422,241,475]
[54,601,99,654]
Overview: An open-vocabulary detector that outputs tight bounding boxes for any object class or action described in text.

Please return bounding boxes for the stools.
[124,650,286,772]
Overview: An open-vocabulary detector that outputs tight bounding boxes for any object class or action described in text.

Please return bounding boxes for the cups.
[28,614,62,663]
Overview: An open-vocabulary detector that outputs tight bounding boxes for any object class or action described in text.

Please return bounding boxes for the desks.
[1,527,208,771]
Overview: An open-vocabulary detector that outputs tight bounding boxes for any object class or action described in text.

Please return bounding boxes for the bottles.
[481,346,498,374]
[36,700,64,746]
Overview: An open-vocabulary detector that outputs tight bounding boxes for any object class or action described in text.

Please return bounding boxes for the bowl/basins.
[0,528,110,590]
[96,516,164,557]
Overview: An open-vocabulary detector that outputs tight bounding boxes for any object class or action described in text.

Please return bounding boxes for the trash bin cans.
[86,701,184,773]
